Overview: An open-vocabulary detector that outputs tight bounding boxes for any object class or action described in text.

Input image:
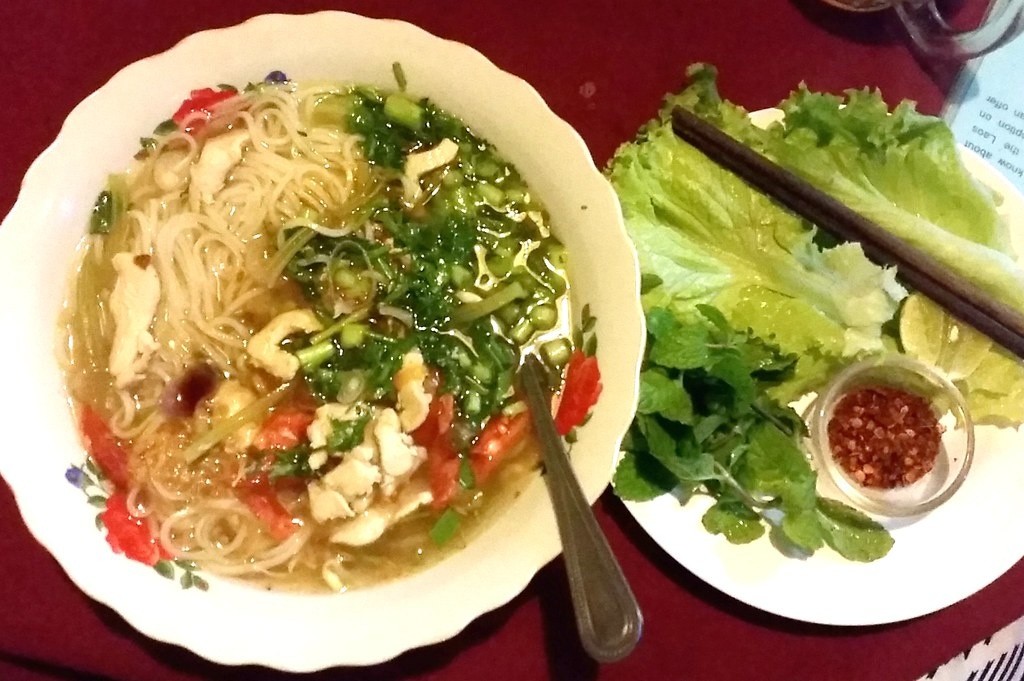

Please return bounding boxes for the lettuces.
[596,64,1023,582]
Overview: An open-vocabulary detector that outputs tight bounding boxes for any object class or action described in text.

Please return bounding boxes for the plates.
[599,108,1024,627]
[0,16,647,673]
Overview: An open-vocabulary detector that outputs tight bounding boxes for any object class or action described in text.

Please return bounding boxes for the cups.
[817,360,975,521]
[832,3,1024,63]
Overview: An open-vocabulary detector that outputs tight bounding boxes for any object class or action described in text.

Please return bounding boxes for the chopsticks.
[667,108,1024,357]
